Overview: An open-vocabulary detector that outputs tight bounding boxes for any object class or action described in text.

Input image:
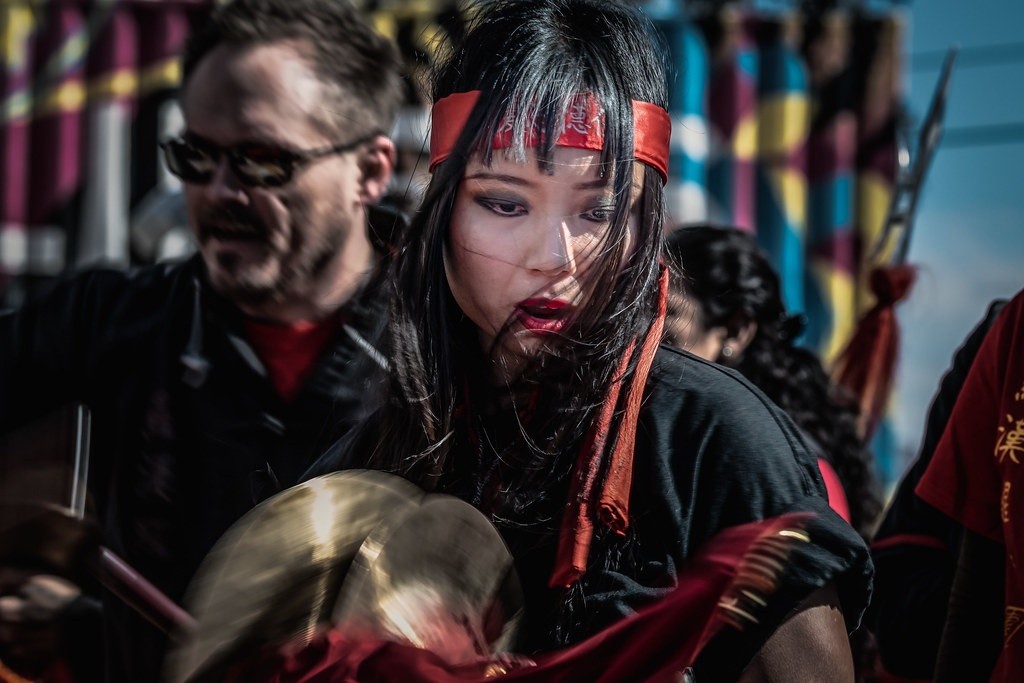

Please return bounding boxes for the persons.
[0,1,419,683]
[852,280,1023,681]
[177,0,882,680]
[657,220,881,584]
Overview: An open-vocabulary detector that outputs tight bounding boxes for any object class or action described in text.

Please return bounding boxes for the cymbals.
[161,468,426,682]
[324,490,523,683]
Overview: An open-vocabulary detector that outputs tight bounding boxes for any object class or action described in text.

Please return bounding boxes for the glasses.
[158,127,389,186]
